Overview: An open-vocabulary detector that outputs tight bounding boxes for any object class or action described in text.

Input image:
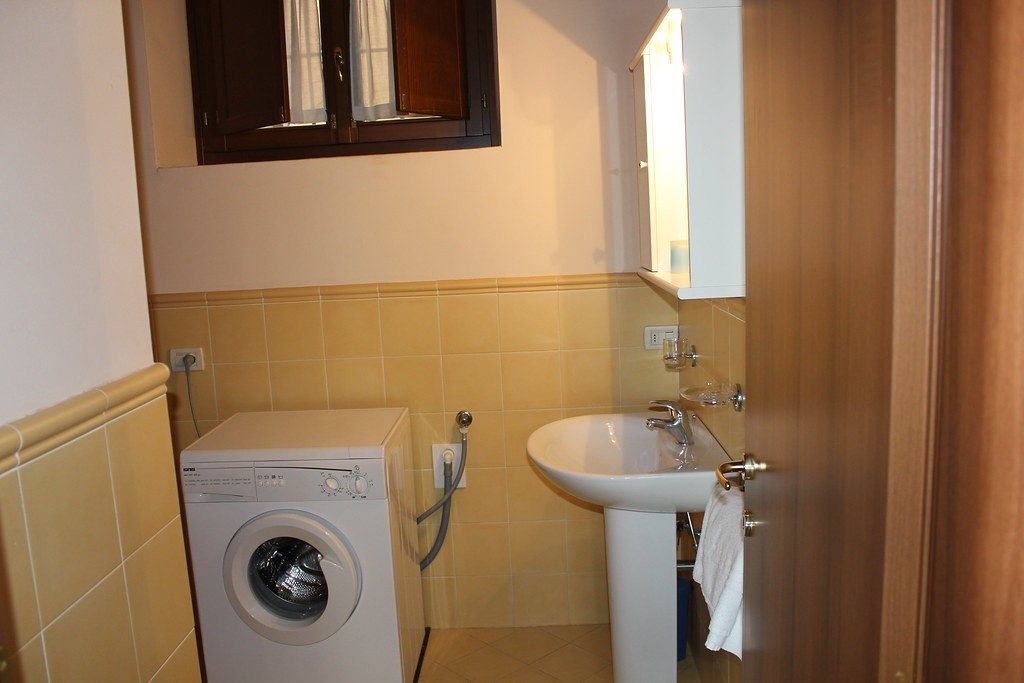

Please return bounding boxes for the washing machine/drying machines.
[180,404,431,682]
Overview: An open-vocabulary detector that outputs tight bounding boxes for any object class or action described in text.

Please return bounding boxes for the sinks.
[525,406,742,514]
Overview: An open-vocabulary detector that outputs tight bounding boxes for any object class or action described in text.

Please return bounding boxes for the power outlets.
[170,348,204,372]
[644,325,679,350]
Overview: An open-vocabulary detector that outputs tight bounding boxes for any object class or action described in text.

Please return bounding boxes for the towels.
[691,479,754,662]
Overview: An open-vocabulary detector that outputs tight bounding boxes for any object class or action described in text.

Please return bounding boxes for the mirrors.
[643,8,688,277]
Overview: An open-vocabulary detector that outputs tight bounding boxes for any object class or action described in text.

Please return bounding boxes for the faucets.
[644,397,696,446]
